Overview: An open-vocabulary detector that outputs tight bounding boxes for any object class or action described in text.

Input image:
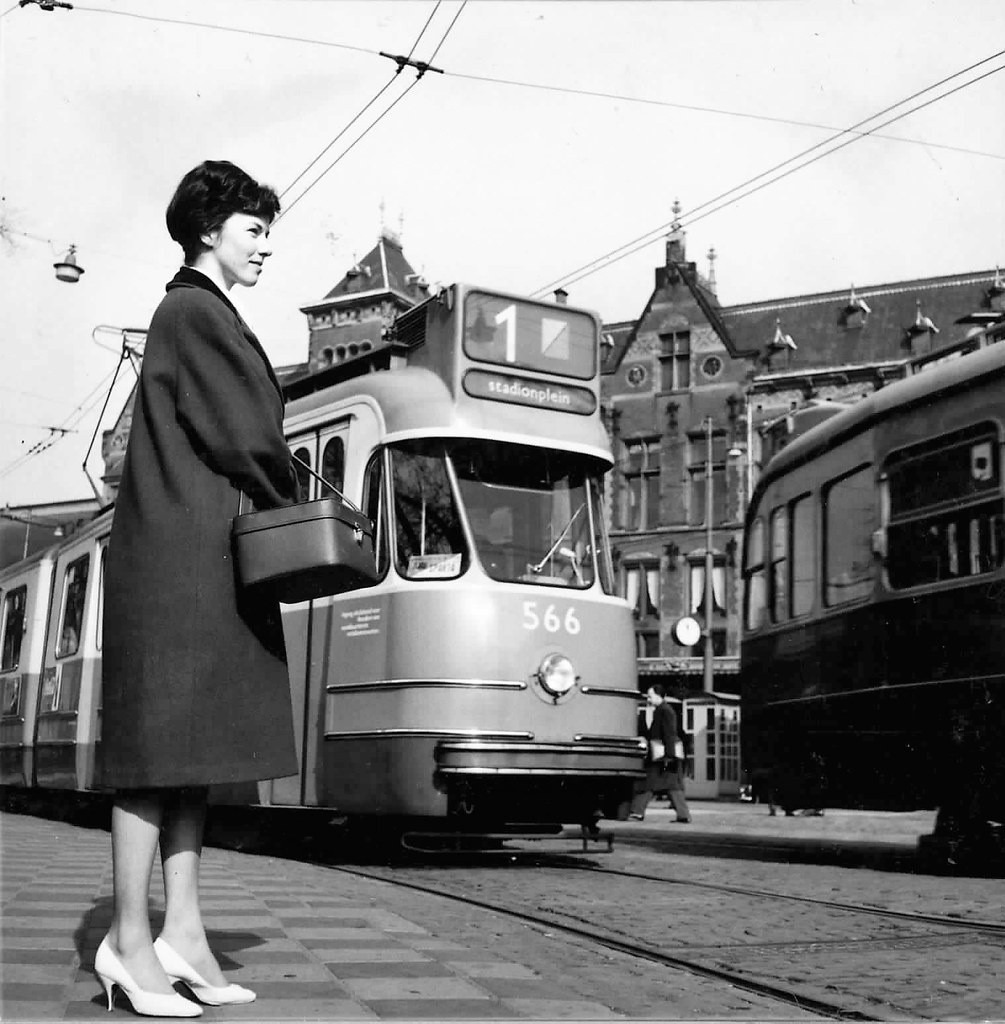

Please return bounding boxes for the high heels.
[153,937,256,1006]
[93,937,203,1018]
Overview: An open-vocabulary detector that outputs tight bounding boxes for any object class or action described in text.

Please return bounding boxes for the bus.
[737,323,1005,867]
[0,282,647,862]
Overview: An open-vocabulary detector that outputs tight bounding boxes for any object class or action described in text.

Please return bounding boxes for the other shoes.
[629,813,645,821]
[670,816,690,823]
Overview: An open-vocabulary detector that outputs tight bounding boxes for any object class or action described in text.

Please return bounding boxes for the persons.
[629,684,692,823]
[93,161,299,1017]
[886,479,951,589]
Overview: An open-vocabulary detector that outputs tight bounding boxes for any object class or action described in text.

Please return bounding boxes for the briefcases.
[233,454,377,604]
